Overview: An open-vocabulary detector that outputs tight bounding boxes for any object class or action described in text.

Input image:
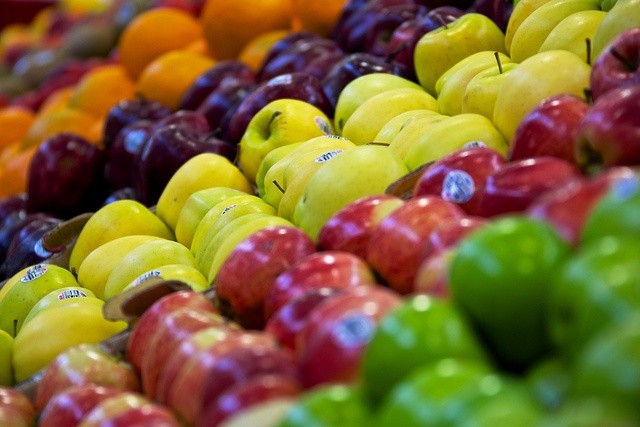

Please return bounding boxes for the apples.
[273,152,356,225]
[290,141,412,243]
[573,319,638,399]
[442,214,577,345]
[508,1,600,65]
[210,223,316,328]
[69,199,177,281]
[187,192,280,266]
[263,135,358,210]
[153,151,255,237]
[100,61,241,204]
[413,143,505,220]
[468,364,630,426]
[0,194,66,283]
[359,295,488,410]
[76,233,198,302]
[478,155,578,218]
[200,212,298,301]
[153,322,296,407]
[589,0,638,64]
[1,383,32,427]
[13,297,129,386]
[404,111,511,177]
[270,381,371,427]
[29,341,141,399]
[197,367,292,427]
[26,131,112,221]
[413,248,459,292]
[251,140,302,201]
[16,284,98,328]
[35,379,117,426]
[541,232,638,349]
[126,287,220,376]
[506,90,585,169]
[491,49,592,147]
[536,9,610,60]
[574,178,639,254]
[312,191,407,255]
[499,0,549,56]
[339,84,441,147]
[1,261,80,339]
[586,26,639,101]
[0,319,18,388]
[318,0,415,74]
[369,105,442,145]
[237,98,334,186]
[80,387,180,427]
[387,0,508,32]
[375,358,501,427]
[387,113,453,165]
[173,186,251,252]
[112,263,210,309]
[527,163,636,246]
[412,10,507,103]
[214,1,322,103]
[459,60,524,123]
[433,47,511,114]
[363,193,464,291]
[574,77,638,183]
[298,287,399,395]
[262,248,374,350]
[329,71,425,137]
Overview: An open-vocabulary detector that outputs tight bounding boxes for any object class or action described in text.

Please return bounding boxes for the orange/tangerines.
[116,1,347,106]
[0,29,134,199]
